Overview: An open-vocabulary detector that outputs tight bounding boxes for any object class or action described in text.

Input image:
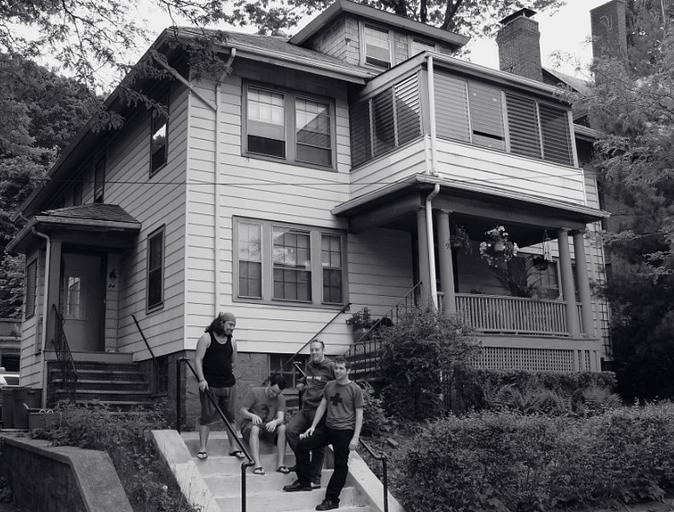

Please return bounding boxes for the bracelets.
[198,379,203,382]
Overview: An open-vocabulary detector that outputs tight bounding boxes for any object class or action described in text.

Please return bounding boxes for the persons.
[193,312,245,460]
[283,356,364,510]
[239,375,286,474]
[285,338,335,488]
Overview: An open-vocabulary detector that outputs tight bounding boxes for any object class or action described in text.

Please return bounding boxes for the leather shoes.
[315,497,339,510]
[282,480,312,492]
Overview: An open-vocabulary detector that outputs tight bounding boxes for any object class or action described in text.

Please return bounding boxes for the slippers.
[252,467,266,474]
[197,452,208,459]
[228,450,246,459]
[275,465,290,474]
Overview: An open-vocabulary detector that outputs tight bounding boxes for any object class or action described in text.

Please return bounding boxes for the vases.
[492,242,506,252]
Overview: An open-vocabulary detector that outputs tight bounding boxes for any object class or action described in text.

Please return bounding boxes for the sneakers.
[311,482,322,489]
[288,465,297,472]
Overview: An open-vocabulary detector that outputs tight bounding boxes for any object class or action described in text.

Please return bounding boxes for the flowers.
[480,225,520,269]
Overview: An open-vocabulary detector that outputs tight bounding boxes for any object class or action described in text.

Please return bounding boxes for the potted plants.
[347,307,375,342]
